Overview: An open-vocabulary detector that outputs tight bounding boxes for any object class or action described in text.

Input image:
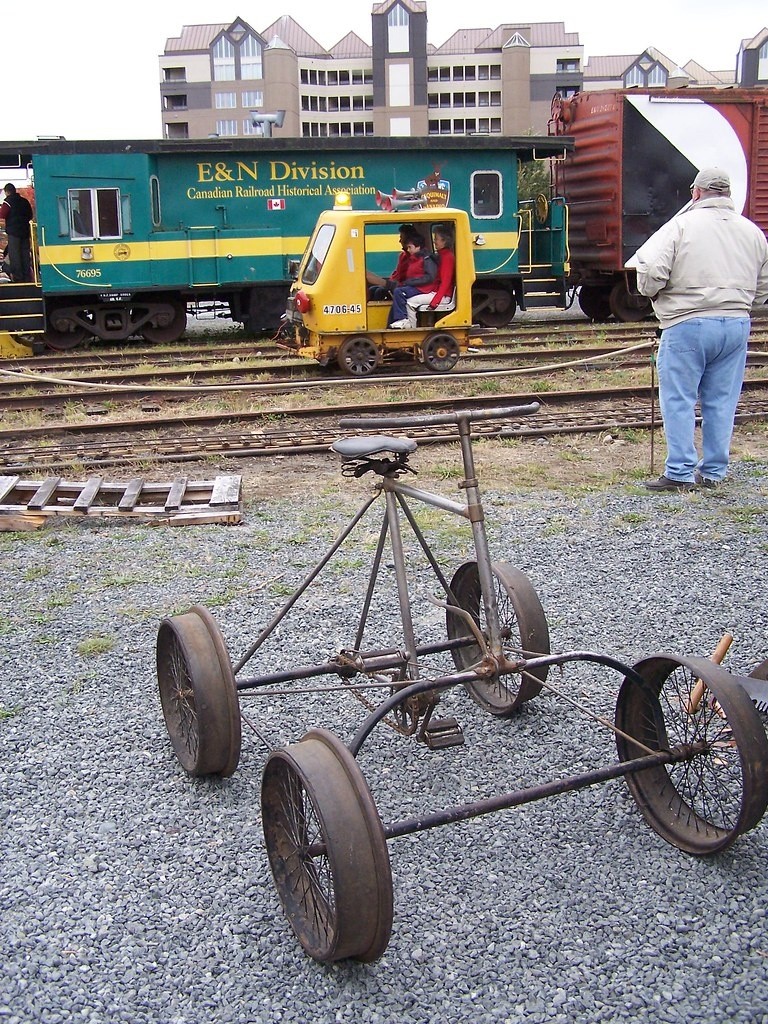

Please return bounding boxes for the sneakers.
[645,474,695,491]
[695,472,717,487]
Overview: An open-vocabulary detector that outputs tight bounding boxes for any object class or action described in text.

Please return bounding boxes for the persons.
[0,183,33,286]
[636,165,768,491]
[365,224,456,330]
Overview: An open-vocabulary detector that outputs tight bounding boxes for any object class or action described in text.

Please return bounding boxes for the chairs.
[416,286,456,312]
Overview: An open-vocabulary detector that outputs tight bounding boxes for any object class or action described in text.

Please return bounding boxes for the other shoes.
[389,318,410,328]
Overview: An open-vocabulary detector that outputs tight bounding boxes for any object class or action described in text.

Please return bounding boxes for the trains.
[0,88,768,358]
[274,188,478,378]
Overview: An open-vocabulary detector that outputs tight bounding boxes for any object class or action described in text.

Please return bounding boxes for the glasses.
[690,189,695,195]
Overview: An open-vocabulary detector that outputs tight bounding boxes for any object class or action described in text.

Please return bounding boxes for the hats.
[689,167,730,194]
